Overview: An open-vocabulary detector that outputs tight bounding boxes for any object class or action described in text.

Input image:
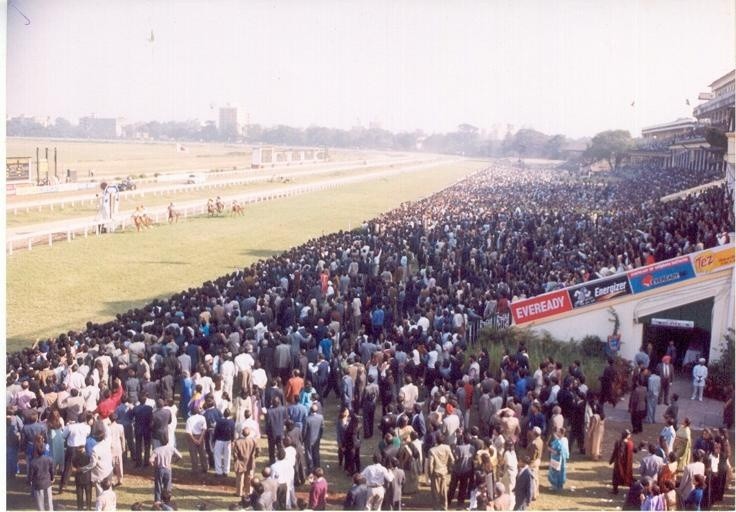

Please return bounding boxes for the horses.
[130,213,156,233]
[216,202,225,212]
[168,207,183,222]
[230,204,245,216]
[206,202,217,217]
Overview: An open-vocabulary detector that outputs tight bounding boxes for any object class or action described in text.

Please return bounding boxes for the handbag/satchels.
[549,458,561,471]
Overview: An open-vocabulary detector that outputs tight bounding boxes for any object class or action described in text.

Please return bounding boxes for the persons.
[249,156,734,283]
[208,195,244,217]
[132,202,179,229]
[6,266,735,509]
